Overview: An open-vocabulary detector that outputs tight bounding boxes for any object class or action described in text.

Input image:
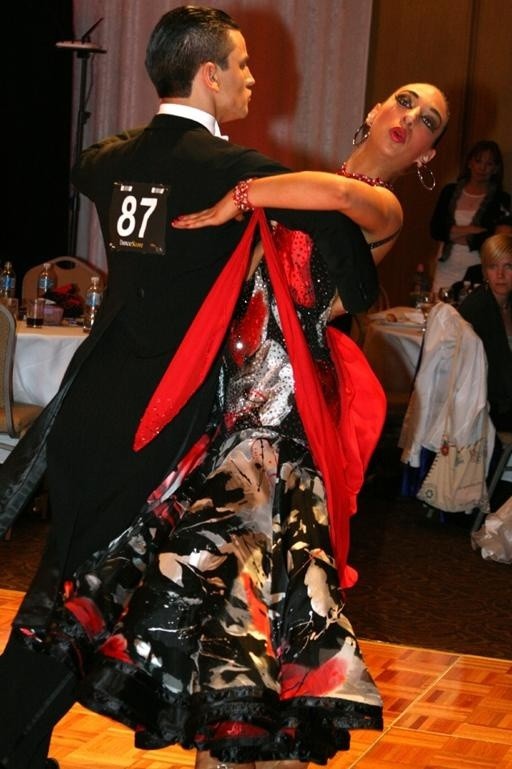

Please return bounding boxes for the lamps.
[54,18,107,267]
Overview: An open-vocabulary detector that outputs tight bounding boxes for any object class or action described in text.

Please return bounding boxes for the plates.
[377,312,419,327]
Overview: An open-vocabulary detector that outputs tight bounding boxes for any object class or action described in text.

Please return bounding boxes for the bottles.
[413,264,424,308]
[82,276,102,333]
[458,281,470,306]
[1,260,18,299]
[37,261,58,295]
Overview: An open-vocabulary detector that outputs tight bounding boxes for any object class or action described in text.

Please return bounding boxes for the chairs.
[397,302,512,534]
[0,305,20,466]
[20,256,106,307]
[369,284,389,313]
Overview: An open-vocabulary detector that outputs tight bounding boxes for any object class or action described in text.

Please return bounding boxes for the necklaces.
[336,161,396,194]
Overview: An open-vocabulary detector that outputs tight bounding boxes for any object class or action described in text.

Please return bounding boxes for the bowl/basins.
[44,304,66,327]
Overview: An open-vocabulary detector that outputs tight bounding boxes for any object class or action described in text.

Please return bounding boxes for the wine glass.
[418,291,436,335]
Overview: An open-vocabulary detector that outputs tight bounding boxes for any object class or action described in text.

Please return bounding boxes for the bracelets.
[234,175,255,211]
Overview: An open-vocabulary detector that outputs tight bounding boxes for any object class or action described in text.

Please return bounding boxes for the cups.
[439,287,453,306]
[24,297,47,327]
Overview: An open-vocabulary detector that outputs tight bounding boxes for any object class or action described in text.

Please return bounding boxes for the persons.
[0,4,378,769]
[12,81,449,769]
[428,139,511,301]
[455,233,512,459]
